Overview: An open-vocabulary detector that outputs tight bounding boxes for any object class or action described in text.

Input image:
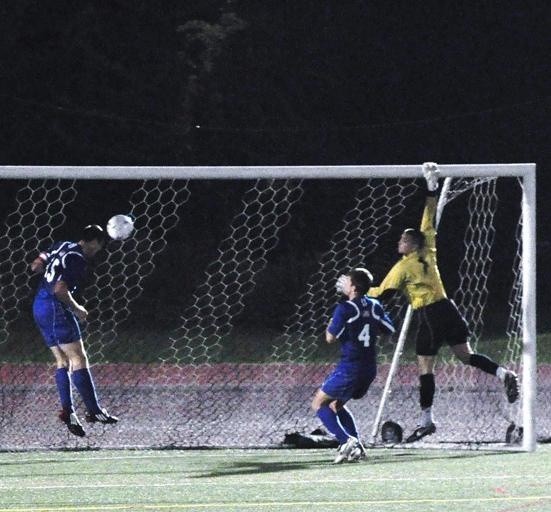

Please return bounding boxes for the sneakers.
[84,408,118,423]
[406,424,436,443]
[504,370,518,403]
[59,409,85,437]
[334,436,367,464]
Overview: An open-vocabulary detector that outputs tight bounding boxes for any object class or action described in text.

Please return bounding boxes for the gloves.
[422,161,440,191]
[334,275,349,292]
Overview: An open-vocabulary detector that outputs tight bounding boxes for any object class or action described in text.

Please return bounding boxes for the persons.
[30,224,121,438]
[335,160,520,445]
[311,266,397,465]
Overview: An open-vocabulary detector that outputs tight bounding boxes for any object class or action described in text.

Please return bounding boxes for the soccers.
[107,214,134,240]
